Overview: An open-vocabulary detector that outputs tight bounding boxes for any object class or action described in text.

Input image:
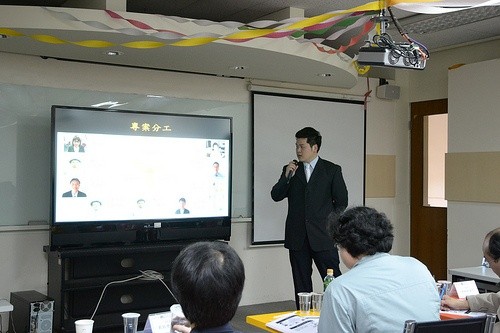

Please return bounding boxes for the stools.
[437,280,451,291]
[0,299,16,333]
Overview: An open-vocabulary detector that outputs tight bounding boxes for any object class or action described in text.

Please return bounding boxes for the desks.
[448,265,500,293]
[49,299,319,333]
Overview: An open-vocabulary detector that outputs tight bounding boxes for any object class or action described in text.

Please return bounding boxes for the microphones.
[286,159,298,184]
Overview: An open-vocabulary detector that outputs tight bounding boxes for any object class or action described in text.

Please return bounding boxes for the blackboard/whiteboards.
[0,81,252,231]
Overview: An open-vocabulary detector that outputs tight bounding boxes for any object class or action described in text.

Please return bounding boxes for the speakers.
[377,85,401,99]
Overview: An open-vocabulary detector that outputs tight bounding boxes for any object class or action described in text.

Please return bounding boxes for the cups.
[75,319,94,333]
[312,292,324,312]
[170,304,188,333]
[436,280,452,298]
[298,293,312,314]
[122,313,141,333]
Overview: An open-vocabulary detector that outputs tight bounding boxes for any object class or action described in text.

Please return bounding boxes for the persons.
[67,138,85,152]
[440,227,500,333]
[210,162,222,177]
[175,198,190,214]
[70,159,80,168]
[171,241,246,333]
[270,127,348,310]
[210,143,222,157]
[91,201,101,210]
[137,200,145,209]
[316,205,441,333]
[62,178,87,197]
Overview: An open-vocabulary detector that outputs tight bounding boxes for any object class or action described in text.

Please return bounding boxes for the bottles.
[323,269,335,292]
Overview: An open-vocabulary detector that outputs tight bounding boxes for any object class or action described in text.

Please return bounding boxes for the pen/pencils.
[440,284,447,300]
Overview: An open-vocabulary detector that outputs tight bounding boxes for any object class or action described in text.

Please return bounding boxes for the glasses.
[484,260,498,268]
[334,242,340,250]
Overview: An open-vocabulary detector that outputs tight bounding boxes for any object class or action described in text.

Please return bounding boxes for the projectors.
[357,46,426,70]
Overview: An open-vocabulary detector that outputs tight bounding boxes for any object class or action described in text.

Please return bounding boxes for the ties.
[304,164,311,183]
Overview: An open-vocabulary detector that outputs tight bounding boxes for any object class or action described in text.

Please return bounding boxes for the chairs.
[403,313,497,333]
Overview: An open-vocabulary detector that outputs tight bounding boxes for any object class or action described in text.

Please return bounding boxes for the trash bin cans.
[0,298,15,333]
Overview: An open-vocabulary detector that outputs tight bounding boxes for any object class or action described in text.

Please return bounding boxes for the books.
[264,313,319,333]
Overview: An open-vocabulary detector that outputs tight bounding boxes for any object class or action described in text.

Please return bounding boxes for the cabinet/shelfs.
[42,241,232,333]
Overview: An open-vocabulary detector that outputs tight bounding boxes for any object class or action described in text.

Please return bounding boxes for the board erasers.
[28,221,49,225]
